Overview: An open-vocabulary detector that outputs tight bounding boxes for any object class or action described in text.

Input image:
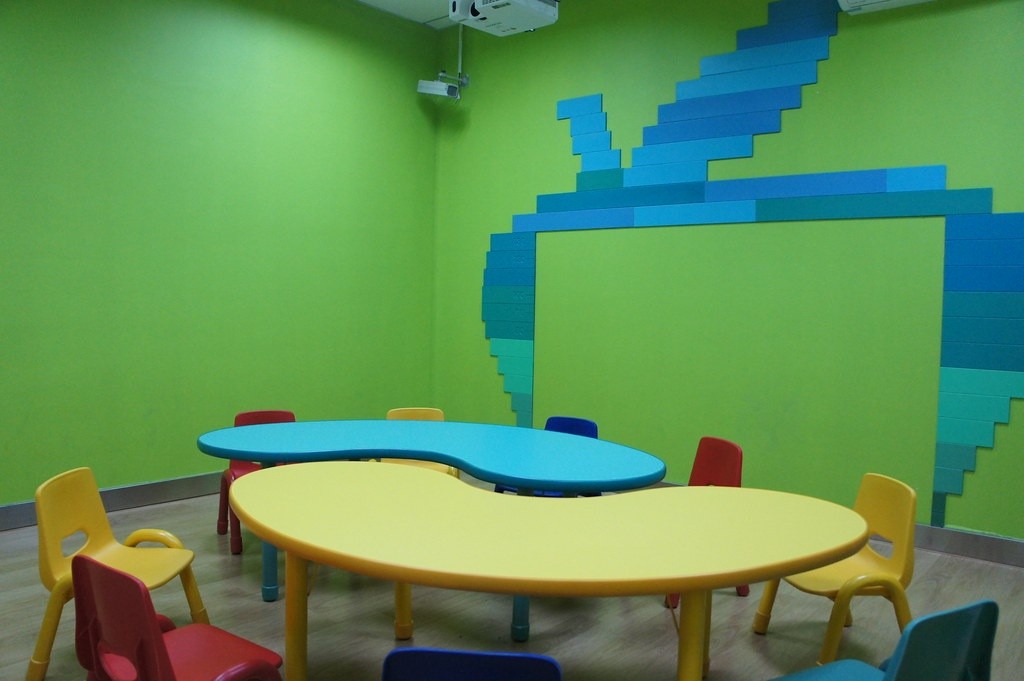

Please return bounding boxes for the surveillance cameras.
[417,79,458,97]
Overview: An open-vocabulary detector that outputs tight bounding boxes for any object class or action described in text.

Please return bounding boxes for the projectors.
[448,0,558,36]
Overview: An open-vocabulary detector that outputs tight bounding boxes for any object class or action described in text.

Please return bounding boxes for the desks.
[197,419,668,644]
[229,461,871,681]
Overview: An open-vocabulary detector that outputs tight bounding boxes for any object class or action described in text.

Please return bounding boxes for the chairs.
[25,466,210,681]
[771,599,1000,681]
[380,646,563,681]
[751,471,918,664]
[216,410,297,557]
[71,554,284,681]
[663,436,749,610]
[497,416,602,500]
[370,407,461,479]
